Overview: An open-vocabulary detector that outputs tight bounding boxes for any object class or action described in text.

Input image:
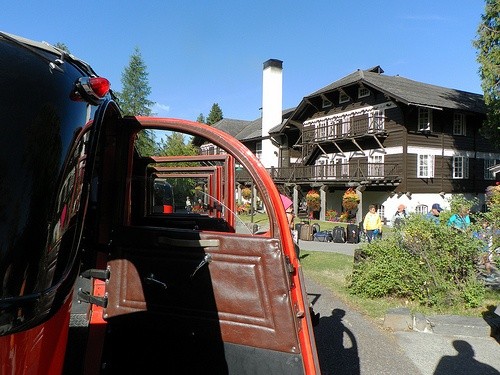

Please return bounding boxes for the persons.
[198,197,202,207]
[424,203,444,225]
[253,194,300,259]
[391,204,409,226]
[185,196,192,213]
[474,221,492,274]
[447,204,471,233]
[363,205,382,243]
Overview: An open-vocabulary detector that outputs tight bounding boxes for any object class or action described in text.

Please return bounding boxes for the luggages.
[301,224,313,241]
[295,224,301,239]
[314,232,332,242]
[347,225,358,243]
[333,225,346,243]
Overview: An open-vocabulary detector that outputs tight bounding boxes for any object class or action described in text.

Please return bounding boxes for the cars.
[0,30,322,375]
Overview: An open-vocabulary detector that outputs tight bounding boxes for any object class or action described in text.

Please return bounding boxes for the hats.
[432,204,443,211]
[398,204,405,210]
